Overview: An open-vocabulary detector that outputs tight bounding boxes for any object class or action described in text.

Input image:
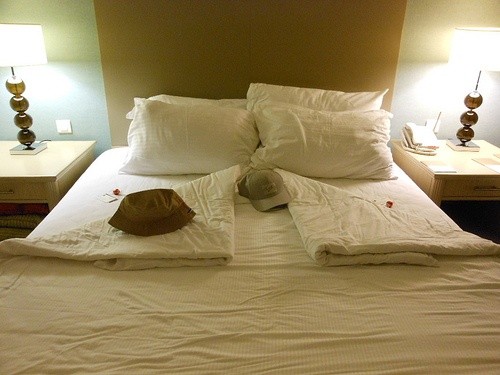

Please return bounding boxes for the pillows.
[126,94,246,119]
[251,96,400,180]
[119,97,259,174]
[247,82,389,124]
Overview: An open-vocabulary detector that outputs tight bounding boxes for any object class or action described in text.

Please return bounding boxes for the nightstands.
[390,139,500,208]
[0,141,97,213]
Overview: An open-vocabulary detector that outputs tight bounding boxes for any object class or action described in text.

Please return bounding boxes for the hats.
[237,168,290,211]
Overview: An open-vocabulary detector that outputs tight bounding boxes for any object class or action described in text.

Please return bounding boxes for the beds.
[0,147,500,375]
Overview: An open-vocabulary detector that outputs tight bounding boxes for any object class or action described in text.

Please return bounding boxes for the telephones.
[402,122,439,148]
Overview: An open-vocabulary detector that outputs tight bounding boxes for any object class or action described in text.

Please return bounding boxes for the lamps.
[447,26,500,152]
[0,22,49,155]
